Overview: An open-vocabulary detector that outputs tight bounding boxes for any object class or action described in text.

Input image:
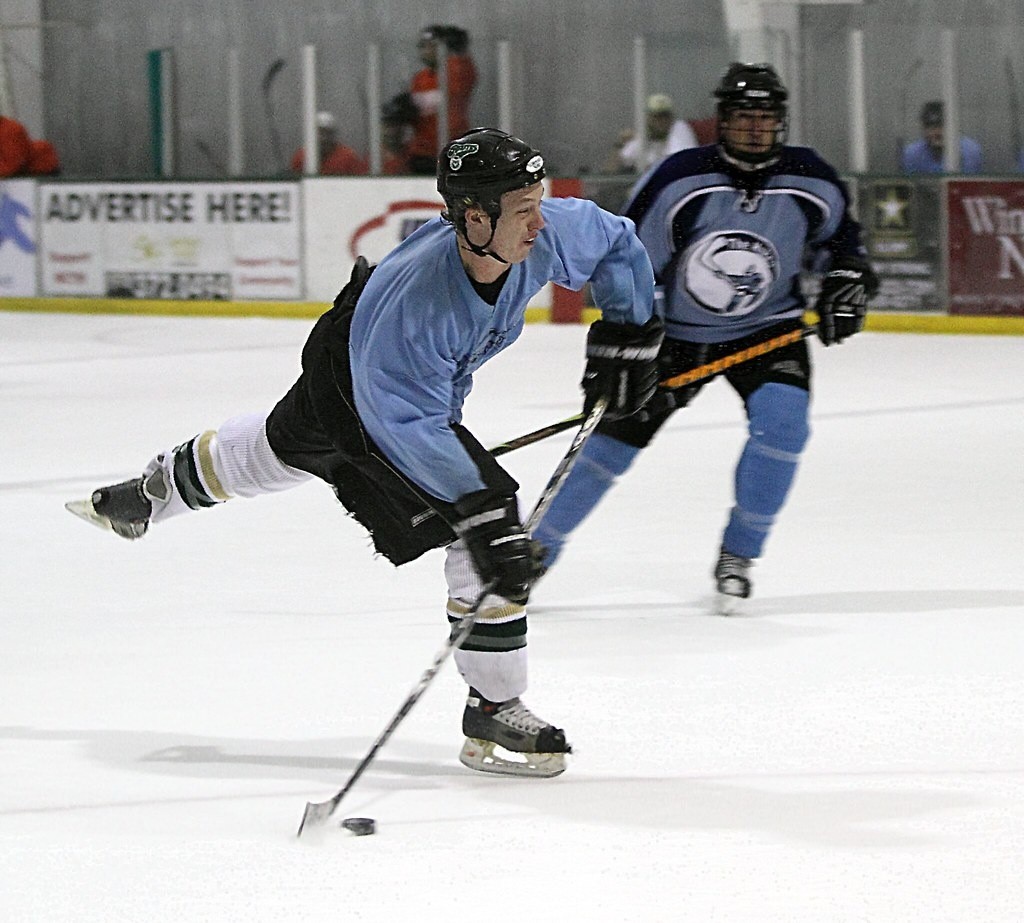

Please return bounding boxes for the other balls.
[341,817,376,836]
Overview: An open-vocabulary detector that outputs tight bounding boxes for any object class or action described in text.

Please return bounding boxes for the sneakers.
[65,478,153,540]
[459,686,572,778]
[713,551,751,614]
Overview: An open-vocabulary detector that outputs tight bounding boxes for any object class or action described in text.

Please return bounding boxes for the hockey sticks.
[296,396,613,839]
[486,324,816,458]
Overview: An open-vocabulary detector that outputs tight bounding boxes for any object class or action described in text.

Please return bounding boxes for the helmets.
[436,127,546,216]
[714,62,790,186]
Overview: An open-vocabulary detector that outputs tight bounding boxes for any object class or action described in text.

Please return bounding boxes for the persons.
[64,126,665,777]
[291,24,478,175]
[599,95,701,177]
[522,62,881,615]
[0,117,60,180]
[903,100,986,175]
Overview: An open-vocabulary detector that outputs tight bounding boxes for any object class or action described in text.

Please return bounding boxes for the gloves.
[451,488,549,605]
[814,263,880,347]
[582,314,667,423]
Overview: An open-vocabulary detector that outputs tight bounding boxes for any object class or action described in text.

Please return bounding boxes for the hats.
[923,102,943,124]
[318,113,334,129]
[645,95,673,113]
[416,25,442,48]
